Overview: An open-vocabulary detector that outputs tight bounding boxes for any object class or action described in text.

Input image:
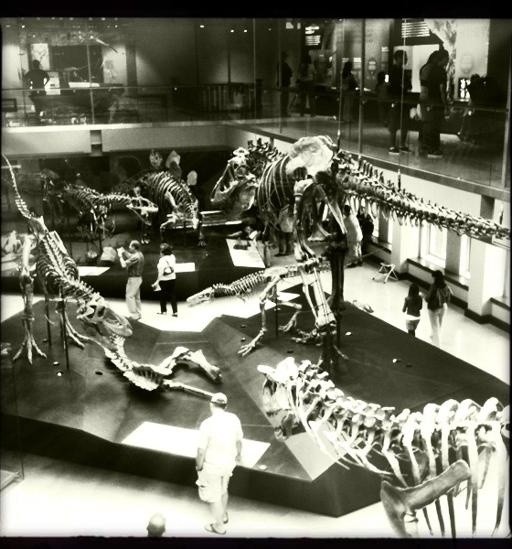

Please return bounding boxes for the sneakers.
[387,146,401,158]
[169,311,181,320]
[128,309,146,323]
[397,143,414,154]
[426,149,446,160]
[344,259,366,269]
[155,309,168,317]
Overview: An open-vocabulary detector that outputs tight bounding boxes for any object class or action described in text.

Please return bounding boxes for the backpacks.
[436,283,453,310]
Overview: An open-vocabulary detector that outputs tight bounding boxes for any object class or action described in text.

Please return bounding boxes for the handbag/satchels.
[162,266,175,278]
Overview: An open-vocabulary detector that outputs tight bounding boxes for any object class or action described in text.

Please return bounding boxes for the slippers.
[200,509,234,536]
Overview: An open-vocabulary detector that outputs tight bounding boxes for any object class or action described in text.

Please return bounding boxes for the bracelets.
[119,256,121,259]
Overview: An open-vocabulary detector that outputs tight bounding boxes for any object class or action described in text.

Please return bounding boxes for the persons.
[117,240,145,320]
[151,243,178,317]
[403,284,422,337]
[194,393,243,535]
[275,197,297,255]
[279,48,486,158]
[426,270,451,347]
[356,206,373,261]
[25,60,49,114]
[343,204,363,267]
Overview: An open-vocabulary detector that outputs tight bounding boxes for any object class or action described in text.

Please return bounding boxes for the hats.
[210,389,230,407]
